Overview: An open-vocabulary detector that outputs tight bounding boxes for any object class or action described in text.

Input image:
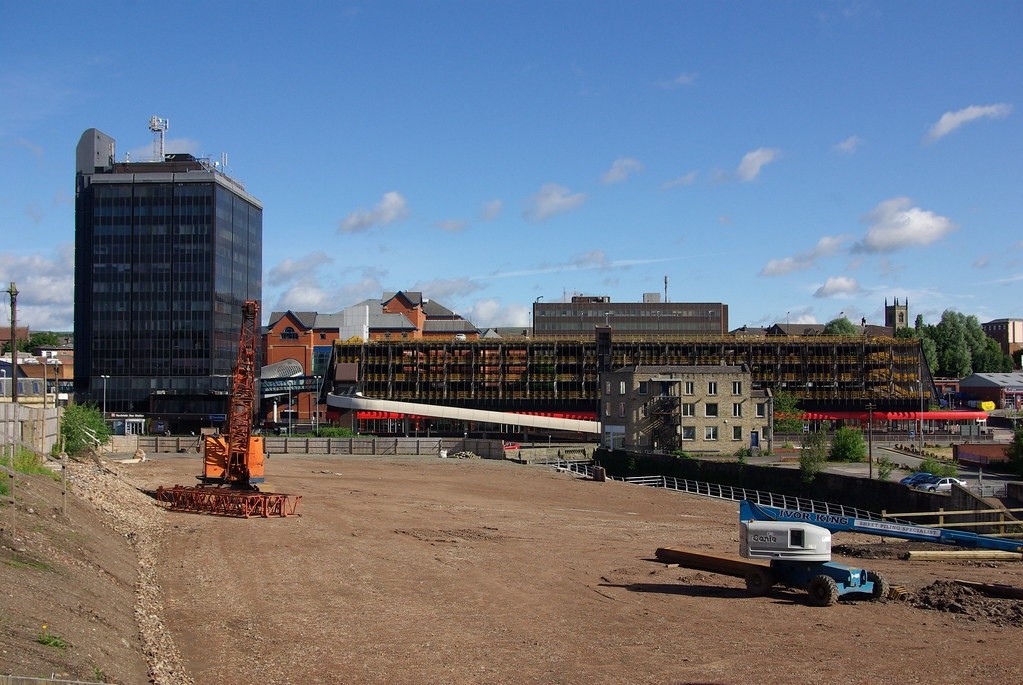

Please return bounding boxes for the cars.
[902,473,939,486]
[492,441,520,450]
[921,477,970,491]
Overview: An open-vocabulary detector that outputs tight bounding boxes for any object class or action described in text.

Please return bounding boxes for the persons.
[165,430,171,437]
[190,431,195,437]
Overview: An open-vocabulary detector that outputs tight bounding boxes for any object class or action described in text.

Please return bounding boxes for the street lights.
[100,376,108,455]
[313,376,323,438]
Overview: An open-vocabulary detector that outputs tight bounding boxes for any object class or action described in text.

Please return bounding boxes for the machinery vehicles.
[739,498,1023,609]
[155,302,304,520]
